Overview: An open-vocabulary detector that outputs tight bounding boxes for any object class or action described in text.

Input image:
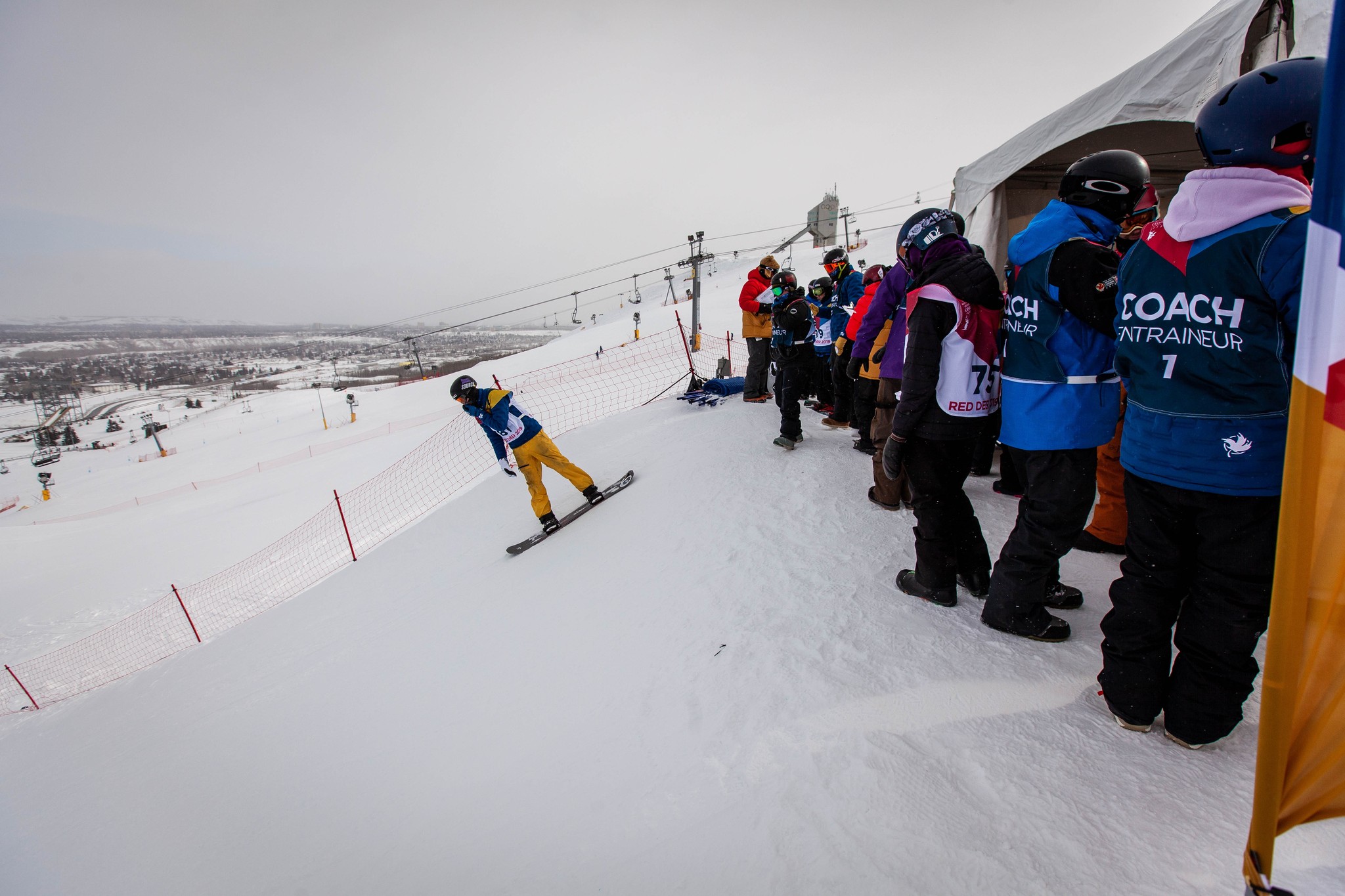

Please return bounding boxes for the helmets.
[1195,55,1327,167]
[808,279,817,292]
[1126,184,1161,217]
[770,150,1151,303]
[450,375,477,400]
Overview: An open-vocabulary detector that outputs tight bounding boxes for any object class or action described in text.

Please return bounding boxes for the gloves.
[758,300,886,380]
[462,404,482,417]
[498,458,517,477]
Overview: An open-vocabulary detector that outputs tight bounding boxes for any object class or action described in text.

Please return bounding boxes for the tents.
[956,0,1334,280]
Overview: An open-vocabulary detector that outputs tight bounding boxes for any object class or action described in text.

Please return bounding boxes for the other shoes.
[1114,716,1151,733]
[1165,729,1204,750]
[1071,529,1126,556]
[743,390,1024,511]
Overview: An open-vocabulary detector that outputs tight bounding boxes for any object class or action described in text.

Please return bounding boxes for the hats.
[757,255,780,278]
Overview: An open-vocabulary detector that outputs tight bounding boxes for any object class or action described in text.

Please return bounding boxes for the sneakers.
[882,433,907,481]
[1044,581,1084,610]
[539,510,560,532]
[582,484,605,505]
[956,572,991,597]
[896,569,957,608]
[981,603,1071,642]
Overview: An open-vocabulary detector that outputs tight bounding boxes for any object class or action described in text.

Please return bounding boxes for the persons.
[805,277,835,414]
[1099,57,1327,750]
[980,150,1152,642]
[1074,183,1161,554]
[883,208,1006,608]
[738,255,780,403]
[845,265,895,455]
[450,375,601,530]
[846,212,966,510]
[771,271,815,449]
[808,248,866,428]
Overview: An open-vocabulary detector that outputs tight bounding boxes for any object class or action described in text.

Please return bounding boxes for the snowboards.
[506,470,634,554]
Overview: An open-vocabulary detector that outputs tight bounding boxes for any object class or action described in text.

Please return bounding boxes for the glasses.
[773,269,779,276]
[1119,205,1160,235]
[824,263,835,273]
[770,286,783,297]
[813,287,824,297]
[455,397,466,404]
[896,257,912,277]
